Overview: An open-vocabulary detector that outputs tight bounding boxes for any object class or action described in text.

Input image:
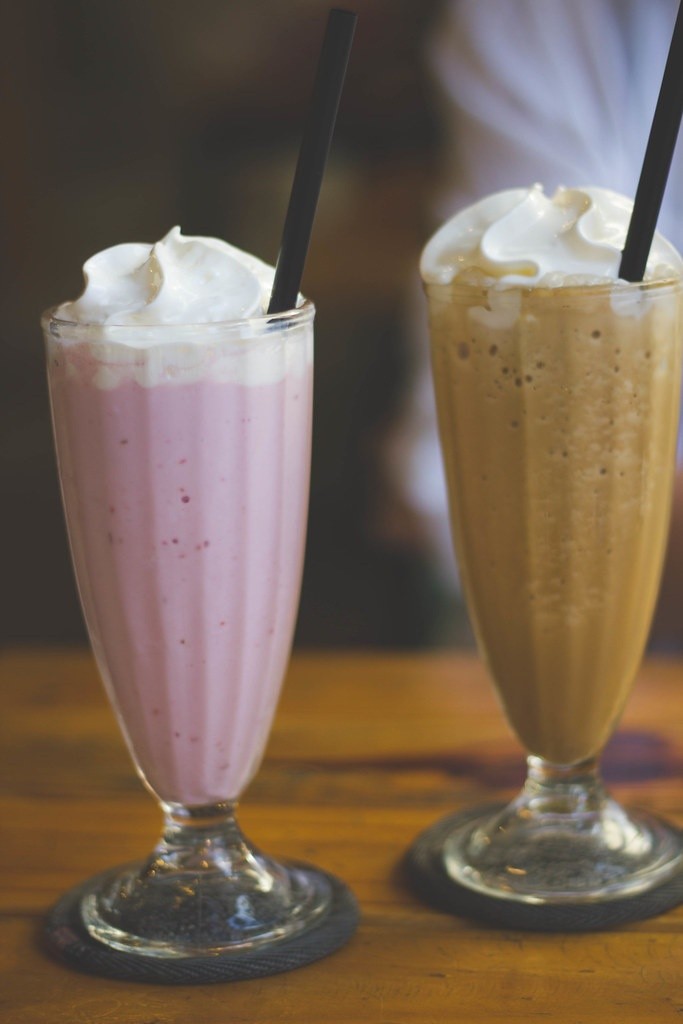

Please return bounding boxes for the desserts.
[418,183,683,765]
[56,225,310,809]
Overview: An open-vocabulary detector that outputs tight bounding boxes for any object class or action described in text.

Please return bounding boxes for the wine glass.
[35,301,358,984]
[397,278,683,934]
